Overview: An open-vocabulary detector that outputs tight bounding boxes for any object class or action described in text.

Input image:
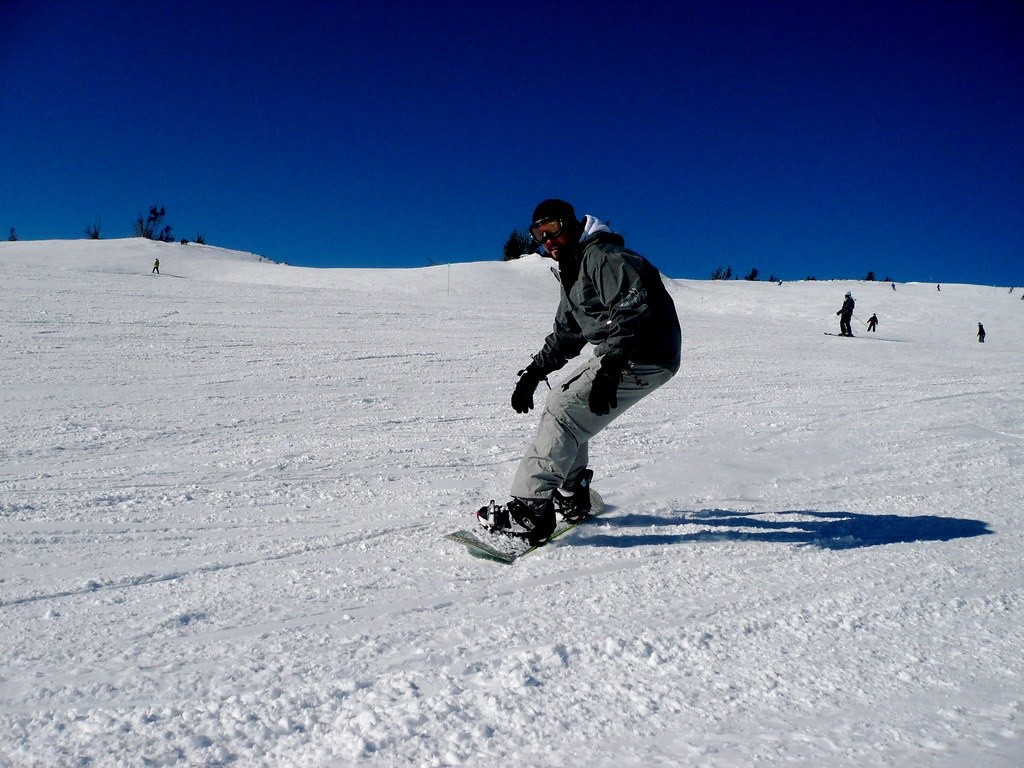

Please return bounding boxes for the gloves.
[511,362,545,413]
[589,356,630,416]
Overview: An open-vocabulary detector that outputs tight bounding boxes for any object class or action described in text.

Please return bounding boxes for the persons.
[478,198,681,545]
[867,313,878,332]
[937,283,940,290]
[977,322,985,343]
[778,279,782,286]
[836,292,854,336]
[1008,286,1014,294]
[891,282,896,290]
[152,258,160,274]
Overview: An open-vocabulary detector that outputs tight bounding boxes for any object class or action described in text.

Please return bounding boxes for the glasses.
[530,218,566,246]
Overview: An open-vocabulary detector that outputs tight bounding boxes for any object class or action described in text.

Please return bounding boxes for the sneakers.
[553,469,594,525]
[477,497,556,547]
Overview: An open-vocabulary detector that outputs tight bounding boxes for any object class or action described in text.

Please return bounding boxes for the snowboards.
[438,482,611,567]
